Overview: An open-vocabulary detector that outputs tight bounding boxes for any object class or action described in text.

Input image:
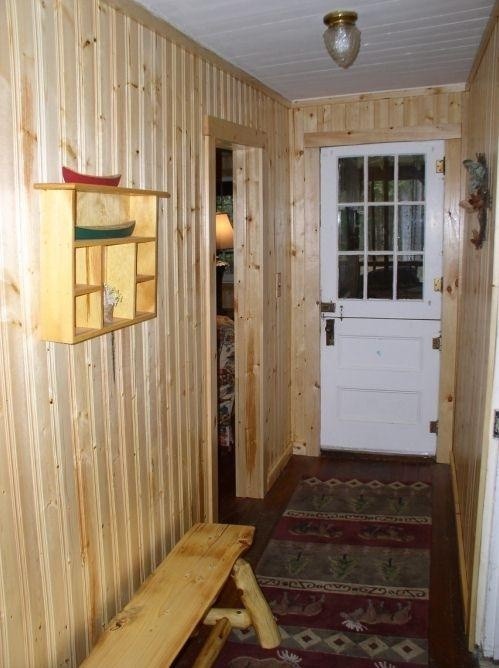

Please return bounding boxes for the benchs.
[70,522,282,668]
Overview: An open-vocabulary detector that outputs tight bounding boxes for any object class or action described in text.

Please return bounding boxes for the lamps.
[216,212,235,254]
[322,9,361,69]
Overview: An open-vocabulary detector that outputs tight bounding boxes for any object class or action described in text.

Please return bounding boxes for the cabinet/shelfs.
[33,182,171,345]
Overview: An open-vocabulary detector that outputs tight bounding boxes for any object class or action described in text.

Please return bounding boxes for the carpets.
[170,474,438,666]
